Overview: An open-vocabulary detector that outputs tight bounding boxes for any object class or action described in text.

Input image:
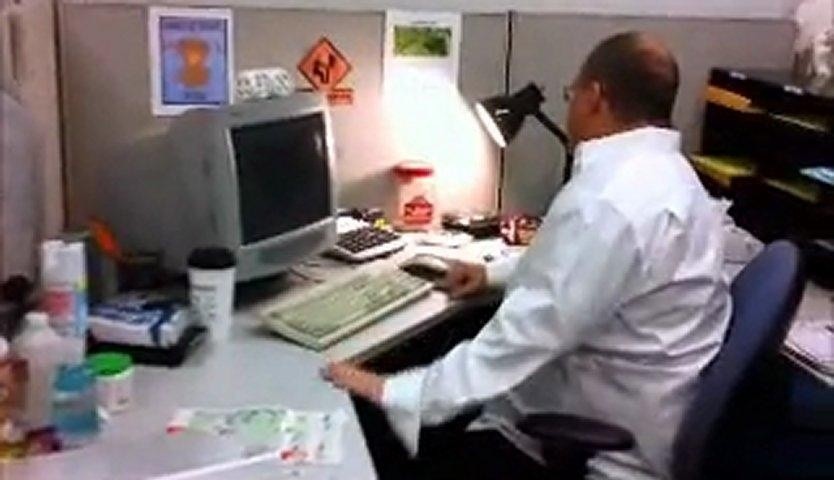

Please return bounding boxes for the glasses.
[562,83,592,104]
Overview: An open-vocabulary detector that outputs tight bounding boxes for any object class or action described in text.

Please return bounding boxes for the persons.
[318,27,735,480]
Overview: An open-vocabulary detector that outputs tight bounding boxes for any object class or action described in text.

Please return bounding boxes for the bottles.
[0,311,98,444]
[391,163,437,230]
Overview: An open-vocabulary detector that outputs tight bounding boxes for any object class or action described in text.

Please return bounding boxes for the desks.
[1,214,533,479]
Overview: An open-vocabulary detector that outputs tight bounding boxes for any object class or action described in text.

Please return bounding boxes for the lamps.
[476,81,571,184]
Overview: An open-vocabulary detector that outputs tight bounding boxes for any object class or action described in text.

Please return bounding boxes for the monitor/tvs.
[117,92,337,302]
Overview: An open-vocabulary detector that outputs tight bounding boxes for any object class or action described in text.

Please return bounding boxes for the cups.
[186,245,238,342]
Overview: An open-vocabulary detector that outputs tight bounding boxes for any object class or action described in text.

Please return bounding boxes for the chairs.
[515,235,813,479]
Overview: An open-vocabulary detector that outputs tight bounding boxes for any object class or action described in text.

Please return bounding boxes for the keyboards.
[324,224,407,262]
[260,258,435,352]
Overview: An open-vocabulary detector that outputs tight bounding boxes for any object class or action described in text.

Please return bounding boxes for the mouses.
[403,255,450,278]
